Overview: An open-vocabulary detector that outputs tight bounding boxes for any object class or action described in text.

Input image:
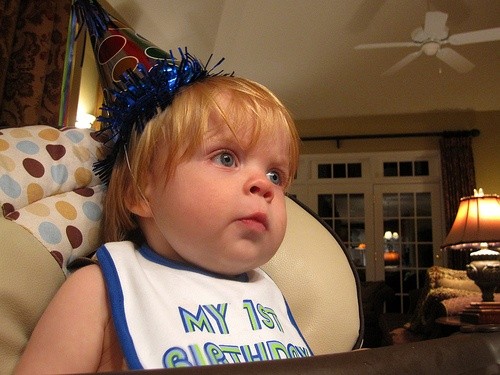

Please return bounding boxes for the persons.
[11,76,314,375]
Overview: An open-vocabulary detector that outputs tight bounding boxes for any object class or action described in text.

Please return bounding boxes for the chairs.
[0,121,364,374]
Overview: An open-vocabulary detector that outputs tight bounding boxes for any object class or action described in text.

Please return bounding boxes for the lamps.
[441,187,500,302]
[422,42,444,75]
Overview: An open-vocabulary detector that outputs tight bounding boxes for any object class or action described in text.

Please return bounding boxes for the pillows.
[403,266,482,332]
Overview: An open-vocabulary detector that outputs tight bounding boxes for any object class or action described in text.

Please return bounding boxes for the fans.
[354,0,500,79]
